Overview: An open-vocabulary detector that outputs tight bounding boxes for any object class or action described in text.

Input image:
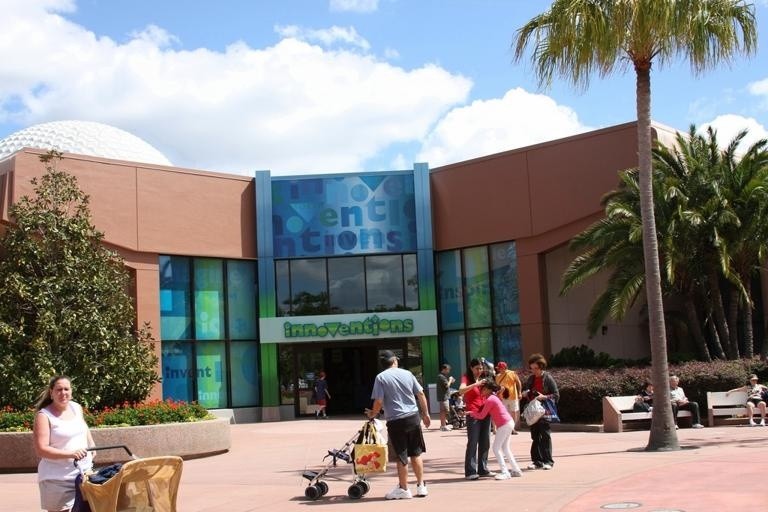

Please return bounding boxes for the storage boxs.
[602,394,694,433]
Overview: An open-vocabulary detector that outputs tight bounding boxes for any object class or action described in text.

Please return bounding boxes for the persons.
[669,375,704,430]
[454,356,523,481]
[523,352,560,470]
[364,349,432,501]
[314,372,331,420]
[436,363,453,431]
[33,374,97,512]
[725,373,768,427]
[633,380,654,412]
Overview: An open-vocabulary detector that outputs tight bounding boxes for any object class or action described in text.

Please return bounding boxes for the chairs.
[465,474,480,480]
[478,471,498,477]
[511,471,523,477]
[494,472,511,480]
[760,419,765,426]
[315,410,319,419]
[385,484,412,500]
[417,481,428,496]
[440,426,451,431]
[675,425,679,430]
[528,463,538,469]
[322,416,330,419]
[691,423,705,428]
[543,463,552,470]
[749,418,756,426]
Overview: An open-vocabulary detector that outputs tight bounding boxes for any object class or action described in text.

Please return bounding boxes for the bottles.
[319,372,327,377]
[494,362,508,369]
[378,350,400,361]
[750,374,759,381]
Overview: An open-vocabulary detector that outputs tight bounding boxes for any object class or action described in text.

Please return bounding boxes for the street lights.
[522,398,546,427]
[542,398,561,424]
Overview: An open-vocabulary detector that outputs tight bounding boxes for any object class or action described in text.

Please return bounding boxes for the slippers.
[353,443,388,475]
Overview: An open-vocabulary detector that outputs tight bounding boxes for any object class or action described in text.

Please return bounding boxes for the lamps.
[706,391,767,427]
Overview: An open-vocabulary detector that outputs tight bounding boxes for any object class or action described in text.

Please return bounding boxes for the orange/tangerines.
[299,411,389,502]
[447,387,468,429]
[70,444,183,512]
